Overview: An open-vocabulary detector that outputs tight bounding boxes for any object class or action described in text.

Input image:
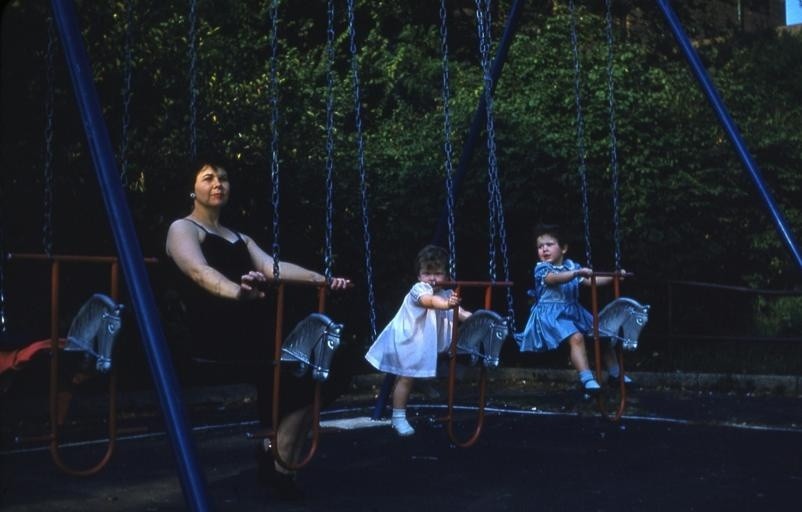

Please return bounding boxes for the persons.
[389,243,474,439]
[162,151,354,491]
[526,227,639,394]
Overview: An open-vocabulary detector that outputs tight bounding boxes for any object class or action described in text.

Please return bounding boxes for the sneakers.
[257,443,303,504]
[390,416,416,436]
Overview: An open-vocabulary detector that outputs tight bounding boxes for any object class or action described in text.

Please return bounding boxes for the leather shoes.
[581,377,604,394]
[608,375,635,386]
